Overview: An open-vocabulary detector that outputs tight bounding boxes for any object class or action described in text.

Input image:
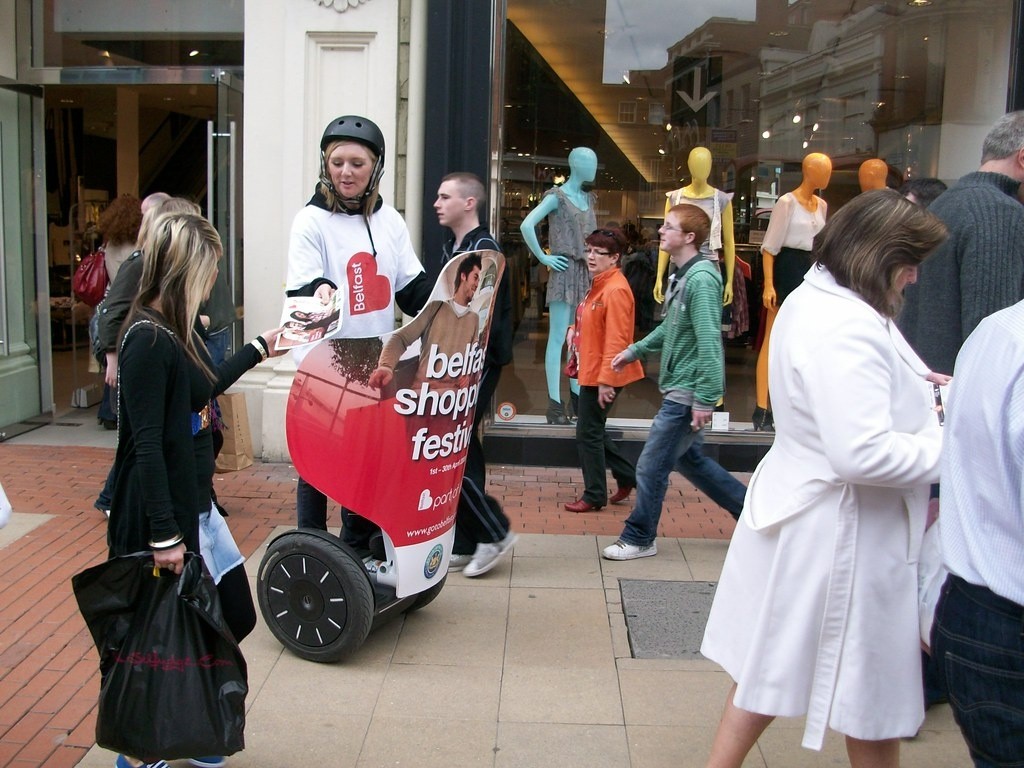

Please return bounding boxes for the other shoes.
[462,529,520,577]
[188,757,225,768]
[114,754,172,768]
[446,553,473,572]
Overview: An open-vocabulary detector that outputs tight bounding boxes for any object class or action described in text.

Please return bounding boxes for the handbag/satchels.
[564,353,579,379]
[88,298,107,367]
[71,233,109,309]
[213,391,253,474]
[72,550,249,760]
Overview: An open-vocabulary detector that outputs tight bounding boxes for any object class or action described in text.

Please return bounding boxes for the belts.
[949,572,1024,622]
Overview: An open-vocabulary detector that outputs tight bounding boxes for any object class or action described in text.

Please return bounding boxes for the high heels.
[97,416,117,430]
[750,405,775,432]
[547,397,575,426]
[609,482,638,504]
[565,499,603,512]
[566,389,580,421]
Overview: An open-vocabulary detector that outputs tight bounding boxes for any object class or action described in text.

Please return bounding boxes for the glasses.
[660,224,689,235]
[592,228,623,254]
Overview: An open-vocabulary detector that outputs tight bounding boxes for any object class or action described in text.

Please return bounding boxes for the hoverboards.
[256,249,507,664]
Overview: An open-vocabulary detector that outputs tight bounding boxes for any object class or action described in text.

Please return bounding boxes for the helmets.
[320,116,385,169]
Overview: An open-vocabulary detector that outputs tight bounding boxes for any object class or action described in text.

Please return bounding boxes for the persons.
[653,147,735,410]
[370,254,482,396]
[520,148,597,423]
[752,153,831,433]
[563,228,645,512]
[900,109,1024,373]
[82,190,289,768]
[412,170,517,575]
[287,114,427,573]
[699,191,949,768]
[615,228,662,412]
[286,311,340,335]
[859,159,888,192]
[601,203,747,561]
[927,297,1024,767]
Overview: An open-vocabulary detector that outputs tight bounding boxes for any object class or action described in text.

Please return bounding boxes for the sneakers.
[602,538,657,559]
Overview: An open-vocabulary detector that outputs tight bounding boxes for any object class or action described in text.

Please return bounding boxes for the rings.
[168,564,175,570]
[609,395,612,397]
